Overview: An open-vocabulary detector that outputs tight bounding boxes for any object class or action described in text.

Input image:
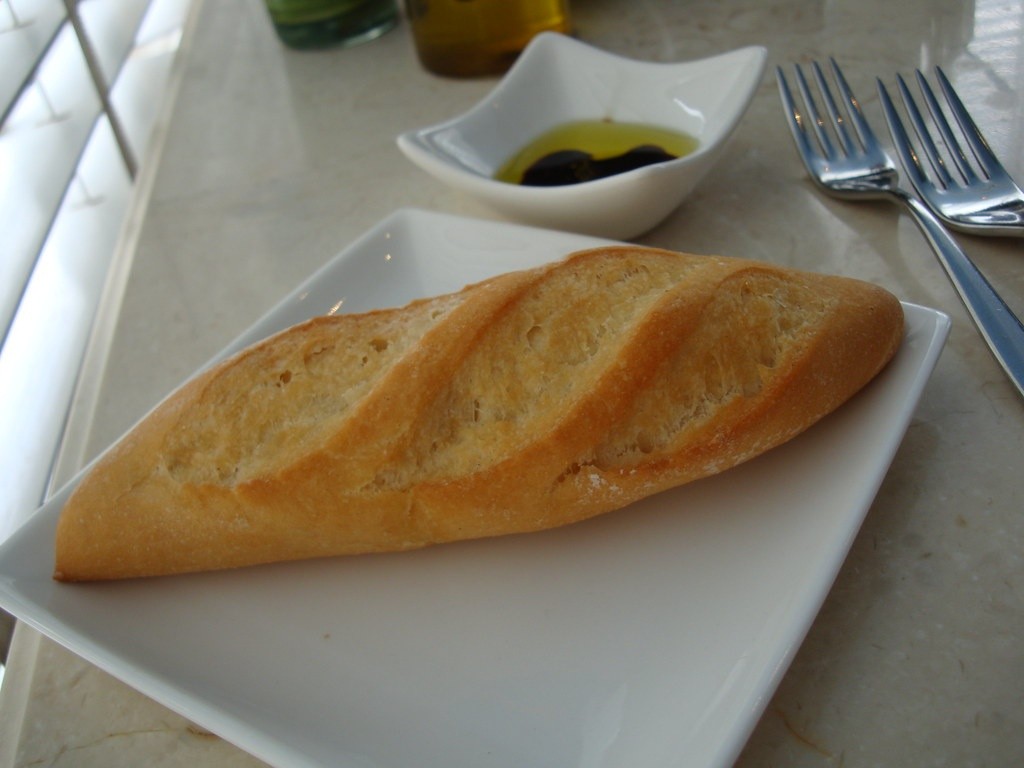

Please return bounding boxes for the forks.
[876,65,1024,237]
[775,56,1024,398]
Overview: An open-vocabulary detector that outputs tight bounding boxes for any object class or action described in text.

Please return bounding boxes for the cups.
[405,0,573,75]
[268,0,398,51]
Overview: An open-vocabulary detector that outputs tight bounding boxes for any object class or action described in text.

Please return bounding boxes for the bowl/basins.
[399,30,768,240]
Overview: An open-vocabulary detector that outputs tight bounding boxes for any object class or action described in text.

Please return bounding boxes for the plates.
[0,206,949,768]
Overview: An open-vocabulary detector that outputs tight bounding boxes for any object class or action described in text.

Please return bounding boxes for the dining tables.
[0,0,1024,768]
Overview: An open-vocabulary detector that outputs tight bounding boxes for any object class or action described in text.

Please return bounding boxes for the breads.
[51,246,907,582]
